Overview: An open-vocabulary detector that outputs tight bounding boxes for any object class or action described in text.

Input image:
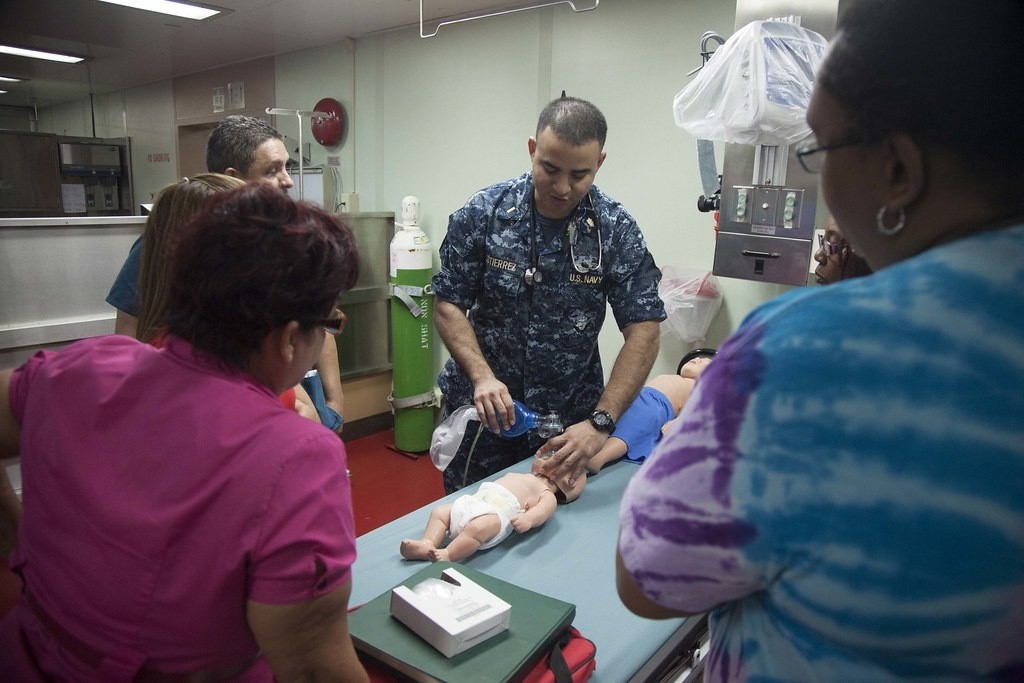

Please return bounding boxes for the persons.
[814,214,873,285]
[105,115,341,434]
[584,354,714,475]
[1,184,371,683]
[432,97,667,495]
[400,454,586,564]
[617,0,1024,683]
[138,173,323,425]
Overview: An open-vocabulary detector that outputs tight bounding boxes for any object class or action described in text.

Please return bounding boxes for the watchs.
[589,409,616,434]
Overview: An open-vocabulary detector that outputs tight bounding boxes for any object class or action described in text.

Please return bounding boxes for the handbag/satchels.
[517,625,598,683]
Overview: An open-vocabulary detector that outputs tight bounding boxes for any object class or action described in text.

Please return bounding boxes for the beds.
[348,446,710,683]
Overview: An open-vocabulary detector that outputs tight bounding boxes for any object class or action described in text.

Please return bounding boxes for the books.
[347,561,576,683]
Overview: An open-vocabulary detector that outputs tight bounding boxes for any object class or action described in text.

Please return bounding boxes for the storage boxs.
[390,566,513,659]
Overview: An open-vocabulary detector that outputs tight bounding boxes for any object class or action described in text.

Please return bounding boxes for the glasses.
[795,133,886,172]
[264,309,345,337]
[818,234,846,257]
